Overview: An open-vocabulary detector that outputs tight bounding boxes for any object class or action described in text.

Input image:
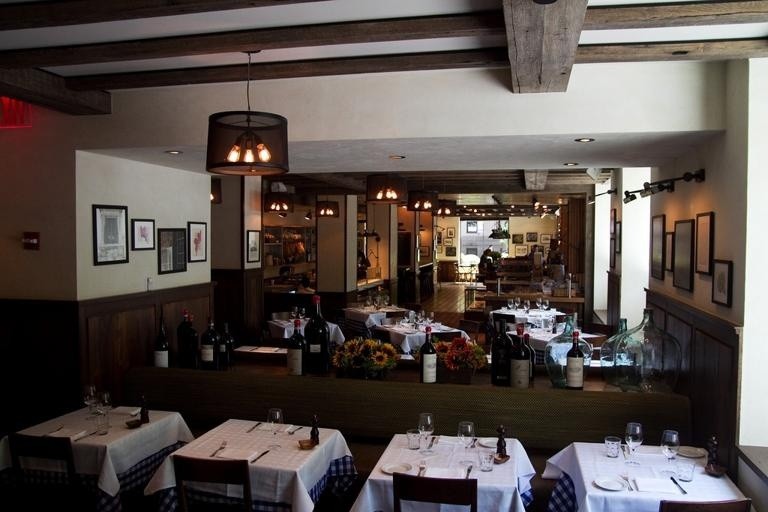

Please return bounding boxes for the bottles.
[139,396,150,424]
[265,232,276,243]
[304,295,330,373]
[566,331,584,390]
[286,320,308,377]
[153,307,234,370]
[488,319,535,388]
[495,424,507,457]
[705,435,720,473]
[418,327,438,385]
[309,413,319,446]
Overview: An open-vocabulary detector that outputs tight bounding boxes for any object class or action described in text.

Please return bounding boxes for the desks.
[541,442,757,512]
[265,303,605,367]
[4,399,191,507]
[348,434,534,512]
[143,418,357,512]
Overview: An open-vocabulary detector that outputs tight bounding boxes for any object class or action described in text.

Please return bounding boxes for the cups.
[96,414,109,434]
[604,436,621,457]
[477,449,494,472]
[270,279,275,285]
[407,428,420,450]
[676,458,696,482]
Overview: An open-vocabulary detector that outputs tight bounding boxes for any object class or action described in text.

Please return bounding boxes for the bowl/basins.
[298,439,315,450]
[124,420,141,430]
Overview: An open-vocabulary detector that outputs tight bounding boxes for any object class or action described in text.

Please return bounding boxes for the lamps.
[204,53,289,176]
[264,171,456,240]
[583,169,706,206]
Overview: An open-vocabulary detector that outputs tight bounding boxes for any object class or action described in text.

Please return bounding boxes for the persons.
[477,249,491,274]
[546,239,560,264]
[527,245,538,261]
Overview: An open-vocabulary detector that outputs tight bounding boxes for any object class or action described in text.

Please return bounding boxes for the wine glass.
[624,422,643,469]
[355,293,390,311]
[455,421,475,468]
[417,412,435,456]
[289,305,305,321]
[660,429,678,479]
[506,294,550,314]
[84,384,113,412]
[265,407,285,452]
[394,308,436,329]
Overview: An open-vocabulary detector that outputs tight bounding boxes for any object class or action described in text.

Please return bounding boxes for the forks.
[416,459,426,477]
[620,470,639,492]
[470,439,477,449]
[210,439,228,458]
[287,426,302,436]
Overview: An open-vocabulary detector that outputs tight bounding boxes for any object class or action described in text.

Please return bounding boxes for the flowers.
[331,335,401,373]
[415,338,489,372]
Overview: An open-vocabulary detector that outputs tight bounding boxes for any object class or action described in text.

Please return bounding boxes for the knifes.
[669,475,688,497]
[426,435,435,449]
[465,465,472,479]
[250,449,270,464]
[245,421,262,433]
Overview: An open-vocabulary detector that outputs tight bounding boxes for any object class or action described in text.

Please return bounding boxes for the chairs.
[657,498,751,512]
[391,472,477,512]
[8,432,80,506]
[172,455,254,512]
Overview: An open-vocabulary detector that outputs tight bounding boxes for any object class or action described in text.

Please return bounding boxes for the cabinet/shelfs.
[264,226,317,264]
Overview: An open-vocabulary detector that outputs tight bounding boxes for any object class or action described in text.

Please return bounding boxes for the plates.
[594,475,628,490]
[478,436,498,447]
[379,461,414,475]
[676,445,705,458]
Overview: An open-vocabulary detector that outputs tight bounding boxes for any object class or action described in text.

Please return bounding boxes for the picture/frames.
[442,238,452,245]
[608,207,622,268]
[247,229,259,263]
[91,204,129,265]
[156,228,187,275]
[436,245,442,254]
[446,247,455,256]
[131,219,154,250]
[510,230,553,258]
[187,221,207,263]
[651,212,733,308]
